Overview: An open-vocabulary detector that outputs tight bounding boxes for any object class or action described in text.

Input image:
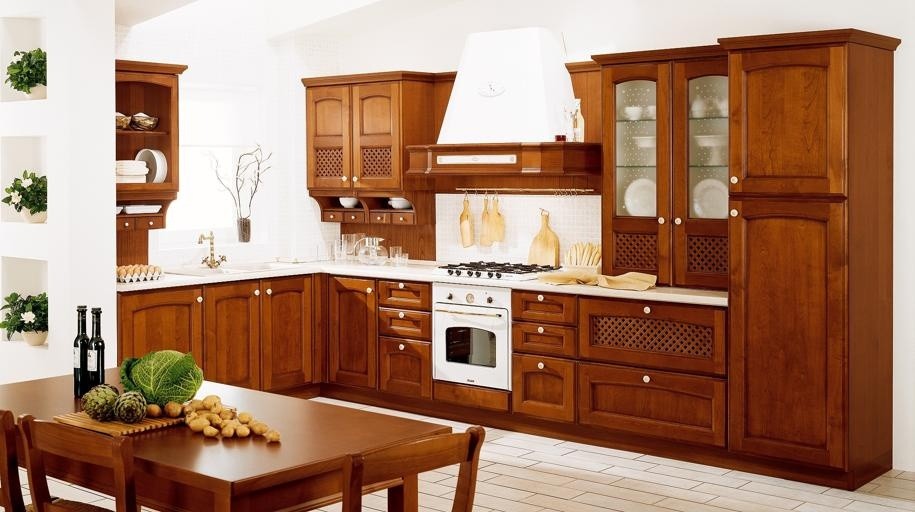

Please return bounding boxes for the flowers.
[0,292,48,341]
[2,169,46,214]
[6,45,45,92]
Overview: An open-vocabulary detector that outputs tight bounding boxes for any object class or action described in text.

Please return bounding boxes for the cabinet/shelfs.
[432,379,511,431]
[327,273,378,408]
[301,70,434,227]
[203,269,321,399]
[716,28,902,201]
[117,284,203,380]
[725,200,893,492]
[378,281,433,417]
[510,289,578,443]
[577,295,726,467]
[116,59,189,231]
[591,44,728,290]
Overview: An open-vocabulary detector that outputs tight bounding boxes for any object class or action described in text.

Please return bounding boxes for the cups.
[314,230,410,268]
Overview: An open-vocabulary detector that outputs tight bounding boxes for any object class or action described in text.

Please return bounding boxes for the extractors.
[402,25,605,179]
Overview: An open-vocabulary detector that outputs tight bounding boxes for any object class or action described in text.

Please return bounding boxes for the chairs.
[16,414,137,512]
[0,409,58,512]
[342,426,485,512]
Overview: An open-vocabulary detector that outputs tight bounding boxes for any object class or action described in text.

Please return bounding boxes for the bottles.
[72,304,90,401]
[566,97,586,144]
[85,308,106,392]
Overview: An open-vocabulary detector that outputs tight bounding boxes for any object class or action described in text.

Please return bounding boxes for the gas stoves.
[432,259,563,282]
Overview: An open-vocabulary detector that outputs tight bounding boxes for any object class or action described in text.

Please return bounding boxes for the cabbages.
[121,349,203,406]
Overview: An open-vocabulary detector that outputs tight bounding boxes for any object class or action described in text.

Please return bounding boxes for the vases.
[29,83,46,99]
[23,330,48,346]
[21,205,47,223]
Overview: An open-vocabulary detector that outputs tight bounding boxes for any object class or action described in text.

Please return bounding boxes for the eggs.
[117,263,162,280]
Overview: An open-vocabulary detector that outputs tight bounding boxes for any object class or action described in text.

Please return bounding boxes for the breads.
[565,242,602,267]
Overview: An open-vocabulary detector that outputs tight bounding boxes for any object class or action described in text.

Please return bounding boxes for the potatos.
[147,395,282,444]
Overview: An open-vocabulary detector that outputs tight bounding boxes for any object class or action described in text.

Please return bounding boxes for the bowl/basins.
[116,116,131,132]
[632,136,655,148]
[339,197,359,210]
[690,96,729,118]
[127,115,159,132]
[386,196,413,210]
[624,104,659,121]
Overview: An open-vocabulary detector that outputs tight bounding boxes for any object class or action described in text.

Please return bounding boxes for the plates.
[116,159,149,176]
[692,179,728,219]
[134,147,168,184]
[624,176,656,218]
[116,205,163,216]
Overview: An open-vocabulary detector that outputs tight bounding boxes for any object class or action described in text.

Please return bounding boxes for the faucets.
[198,231,227,270]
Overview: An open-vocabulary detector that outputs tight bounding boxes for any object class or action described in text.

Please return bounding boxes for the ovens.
[428,281,514,395]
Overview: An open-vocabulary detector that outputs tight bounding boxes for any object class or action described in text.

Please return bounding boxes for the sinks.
[163,263,305,276]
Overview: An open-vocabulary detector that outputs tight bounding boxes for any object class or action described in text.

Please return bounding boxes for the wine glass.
[693,134,728,165]
